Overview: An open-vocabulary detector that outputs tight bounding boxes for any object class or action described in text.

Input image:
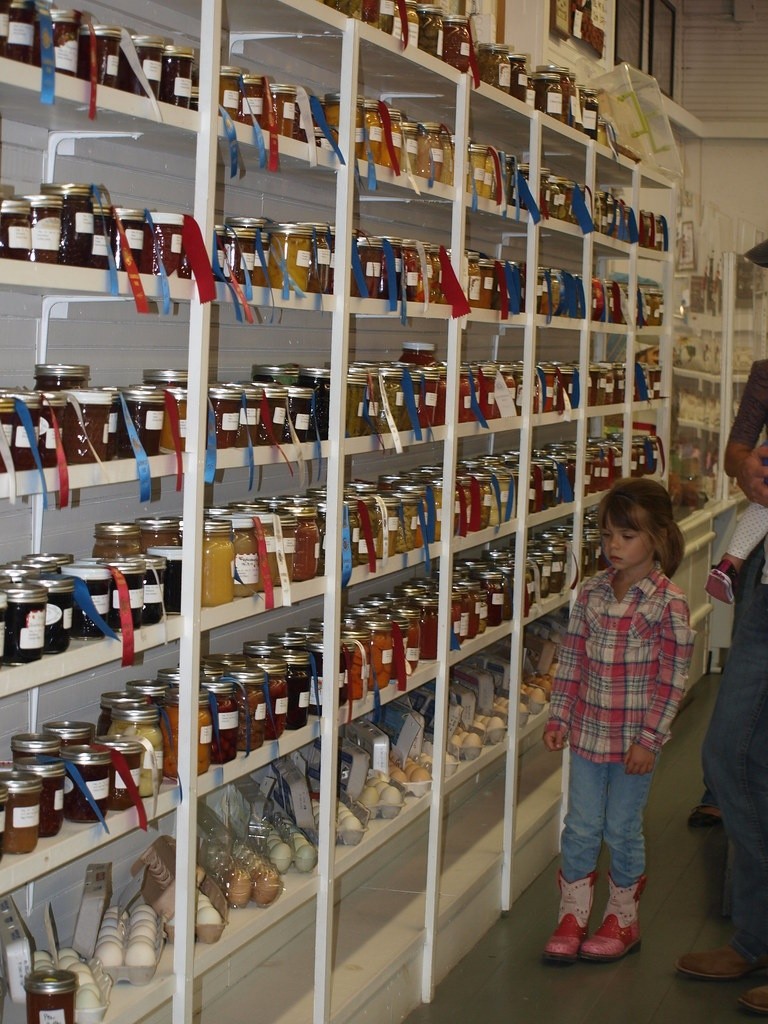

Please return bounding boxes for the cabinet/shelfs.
[672,247,768,673]
[0,0,711,1024]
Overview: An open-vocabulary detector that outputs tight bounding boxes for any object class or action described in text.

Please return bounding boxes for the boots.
[581,868,647,962]
[542,869,598,962]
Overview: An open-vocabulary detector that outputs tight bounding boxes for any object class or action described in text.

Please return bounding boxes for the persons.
[704,503,768,605]
[687,789,724,828]
[677,240,768,1015]
[543,477,698,964]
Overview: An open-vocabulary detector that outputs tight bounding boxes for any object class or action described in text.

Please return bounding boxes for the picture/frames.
[550,0,573,39]
[612,0,677,100]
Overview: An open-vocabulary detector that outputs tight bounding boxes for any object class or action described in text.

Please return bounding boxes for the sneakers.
[704,560,740,604]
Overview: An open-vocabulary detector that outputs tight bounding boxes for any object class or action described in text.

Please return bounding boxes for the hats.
[744,239,768,268]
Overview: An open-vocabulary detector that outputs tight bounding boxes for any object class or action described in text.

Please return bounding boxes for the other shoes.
[688,806,721,827]
[737,985,768,1014]
[674,945,768,977]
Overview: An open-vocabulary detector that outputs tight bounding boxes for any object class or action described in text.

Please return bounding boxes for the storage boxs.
[584,61,684,182]
